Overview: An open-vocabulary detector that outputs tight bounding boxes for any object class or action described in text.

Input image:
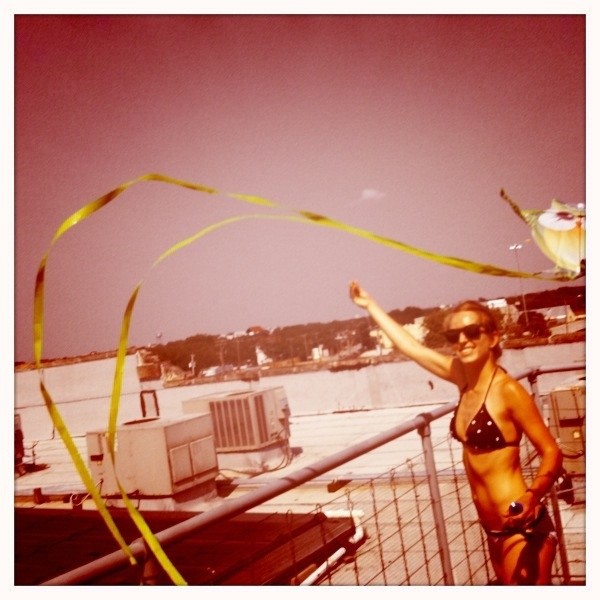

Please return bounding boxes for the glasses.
[445,324,492,343]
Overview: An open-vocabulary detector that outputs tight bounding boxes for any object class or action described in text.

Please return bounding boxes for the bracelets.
[526,487,542,501]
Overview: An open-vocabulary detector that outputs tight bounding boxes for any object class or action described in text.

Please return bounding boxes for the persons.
[348,281,566,584]
